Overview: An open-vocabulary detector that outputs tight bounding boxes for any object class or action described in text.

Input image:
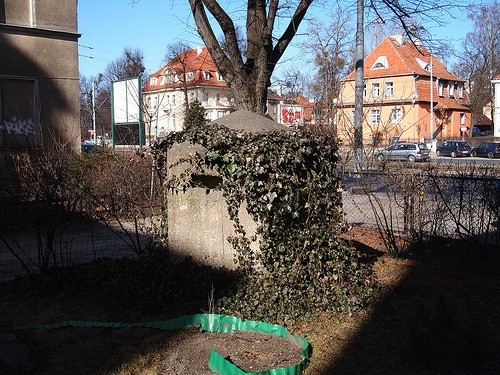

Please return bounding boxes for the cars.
[436,139,471,158]
[471,142,500,159]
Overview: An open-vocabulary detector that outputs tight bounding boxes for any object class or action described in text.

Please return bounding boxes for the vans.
[373,143,430,163]
